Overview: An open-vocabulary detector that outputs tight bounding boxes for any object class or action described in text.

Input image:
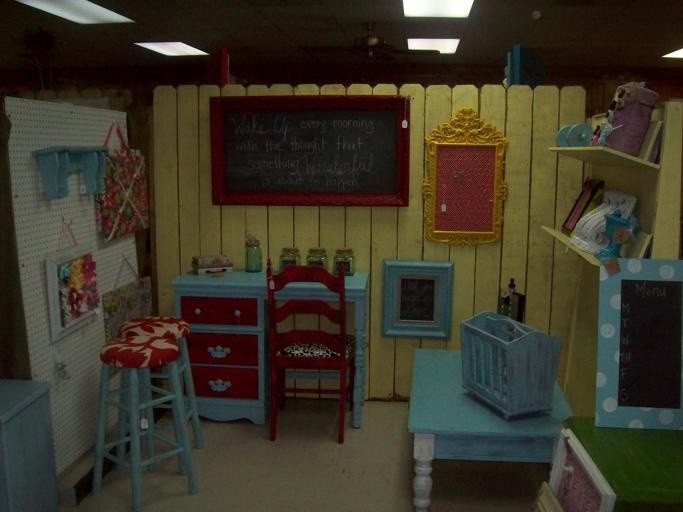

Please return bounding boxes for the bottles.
[245,241,354,276]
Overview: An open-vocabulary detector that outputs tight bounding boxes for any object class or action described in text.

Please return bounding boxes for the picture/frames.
[44,239,102,344]
[381,259,453,339]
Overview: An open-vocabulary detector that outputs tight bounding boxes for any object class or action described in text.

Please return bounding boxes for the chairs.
[263,257,355,444]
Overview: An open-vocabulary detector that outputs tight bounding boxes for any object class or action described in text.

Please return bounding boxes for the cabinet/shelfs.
[538,98,682,416]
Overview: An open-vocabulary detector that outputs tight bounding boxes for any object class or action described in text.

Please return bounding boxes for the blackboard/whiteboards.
[210,94,410,206]
[596,259,682,429]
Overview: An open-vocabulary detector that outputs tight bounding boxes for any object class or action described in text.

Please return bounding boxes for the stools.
[89,315,205,510]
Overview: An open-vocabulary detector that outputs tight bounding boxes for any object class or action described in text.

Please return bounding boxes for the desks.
[407,347,574,512]
[168,268,369,429]
